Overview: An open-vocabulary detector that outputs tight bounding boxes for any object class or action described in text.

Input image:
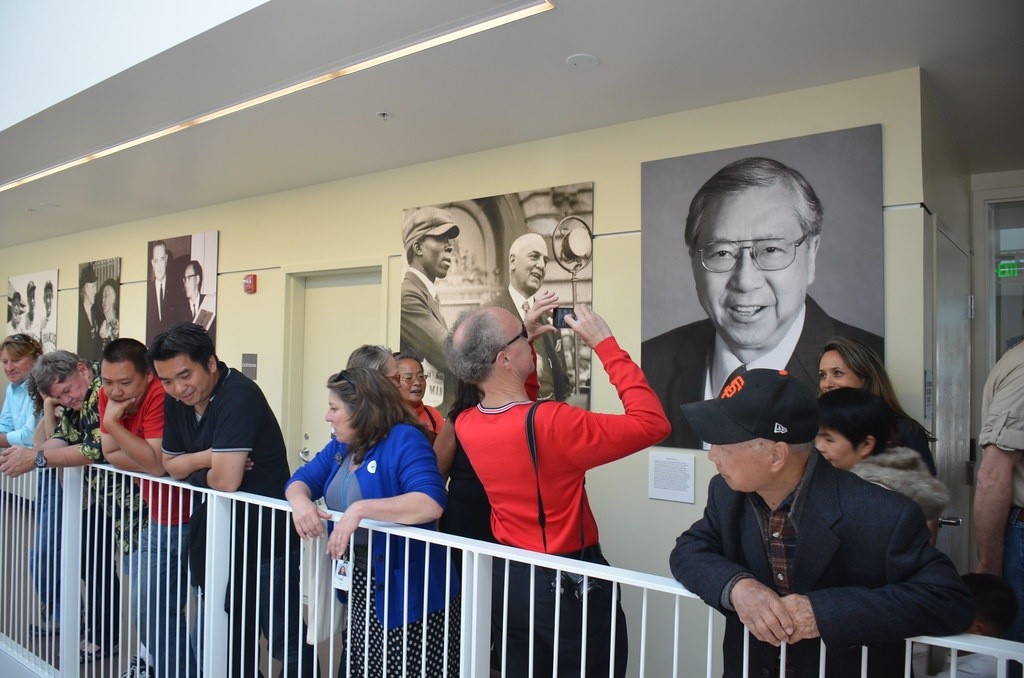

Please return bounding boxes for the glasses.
[694,235,805,272]
[338,370,356,386]
[492,325,528,362]
[13,334,37,349]
[400,374,429,384]
[387,372,401,384]
[182,275,196,283]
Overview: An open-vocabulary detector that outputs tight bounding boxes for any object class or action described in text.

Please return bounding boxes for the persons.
[147,241,217,348]
[641,157,884,451]
[145,321,321,678]
[400,207,573,418]
[0,334,151,678]
[7,281,56,354]
[964,573,1019,678]
[815,386,949,547]
[282,292,672,678]
[76,265,120,362]
[338,566,346,576]
[970,340,1024,678]
[668,368,977,678]
[820,338,938,482]
[98,338,203,678]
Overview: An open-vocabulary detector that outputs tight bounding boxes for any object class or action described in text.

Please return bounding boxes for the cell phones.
[553,308,577,328]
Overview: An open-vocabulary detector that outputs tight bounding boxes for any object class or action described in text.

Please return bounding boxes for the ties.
[435,295,441,310]
[160,284,163,315]
[523,302,530,314]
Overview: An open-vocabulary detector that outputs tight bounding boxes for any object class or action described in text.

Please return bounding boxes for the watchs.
[35,450,46,468]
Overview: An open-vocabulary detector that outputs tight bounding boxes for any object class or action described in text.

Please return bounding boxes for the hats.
[82,266,98,284]
[681,370,820,445]
[45,280,53,290]
[12,292,21,304]
[27,281,36,294]
[401,207,460,252]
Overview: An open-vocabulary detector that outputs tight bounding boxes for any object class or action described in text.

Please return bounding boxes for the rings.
[337,536,342,539]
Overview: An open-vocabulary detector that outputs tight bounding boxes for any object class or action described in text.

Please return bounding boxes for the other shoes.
[79,643,109,665]
[29,622,61,638]
[121,656,154,678]
